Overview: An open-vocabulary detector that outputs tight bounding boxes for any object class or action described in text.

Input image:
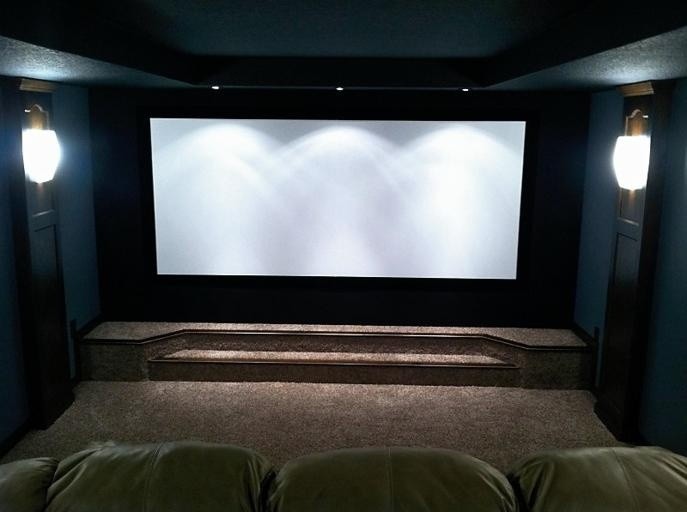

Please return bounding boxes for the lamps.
[22,129,61,184]
[611,134,651,191]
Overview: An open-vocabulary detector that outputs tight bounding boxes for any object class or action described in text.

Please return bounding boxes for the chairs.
[507,446,686,511]
[267,446,518,512]
[46,442,270,510]
[1,457,57,510]
